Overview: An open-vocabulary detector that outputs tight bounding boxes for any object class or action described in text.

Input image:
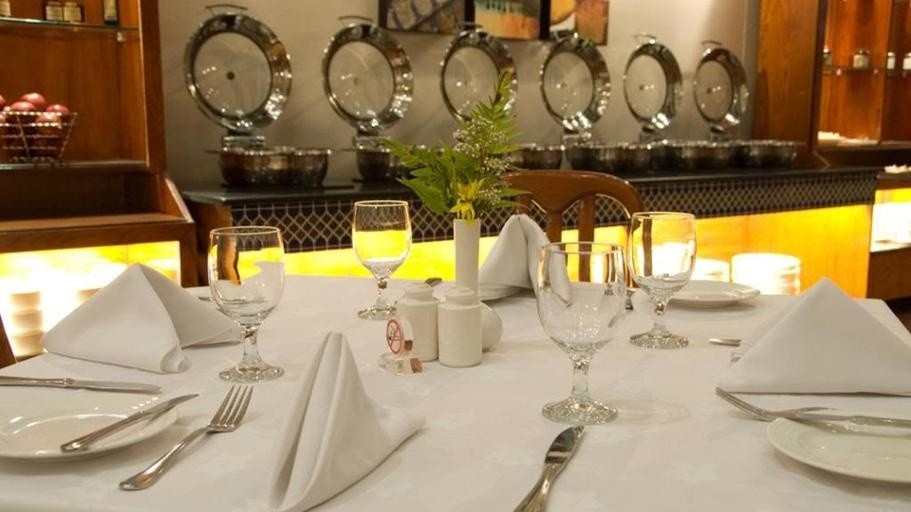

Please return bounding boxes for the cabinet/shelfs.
[0,1,204,365]
[752,0,911,298]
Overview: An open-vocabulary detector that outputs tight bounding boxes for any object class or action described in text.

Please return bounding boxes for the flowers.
[384,68,529,216]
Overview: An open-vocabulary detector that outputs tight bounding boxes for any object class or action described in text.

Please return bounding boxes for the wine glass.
[536,212,697,425]
[351,200,413,321]
[207,226,285,382]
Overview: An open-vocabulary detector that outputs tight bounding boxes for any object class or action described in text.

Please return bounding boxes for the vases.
[452,218,481,302]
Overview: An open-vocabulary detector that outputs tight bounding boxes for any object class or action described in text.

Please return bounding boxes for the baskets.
[0,110,78,169]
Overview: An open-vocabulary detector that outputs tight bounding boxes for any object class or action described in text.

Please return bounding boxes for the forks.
[117,384,252,493]
[714,387,911,432]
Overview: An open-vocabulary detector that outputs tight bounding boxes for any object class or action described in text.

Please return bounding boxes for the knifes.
[60,393,200,453]
[708,339,740,346]
[0,376,163,395]
[511,427,587,512]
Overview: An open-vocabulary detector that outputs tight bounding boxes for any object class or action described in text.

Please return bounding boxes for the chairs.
[502,162,654,283]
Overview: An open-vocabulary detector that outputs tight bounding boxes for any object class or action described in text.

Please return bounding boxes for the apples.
[0,92,69,156]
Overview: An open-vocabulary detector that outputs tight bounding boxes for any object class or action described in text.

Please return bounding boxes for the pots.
[322,15,445,190]
[179,2,334,194]
[623,33,732,168]
[687,38,801,168]
[442,22,566,170]
[537,27,641,173]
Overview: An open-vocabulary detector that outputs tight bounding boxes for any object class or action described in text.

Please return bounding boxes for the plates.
[769,409,911,487]
[0,394,178,462]
[432,278,520,302]
[666,278,761,309]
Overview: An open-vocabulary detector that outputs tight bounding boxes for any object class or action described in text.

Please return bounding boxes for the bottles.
[397,281,502,368]
[823,46,910,69]
[0,0,119,25]
